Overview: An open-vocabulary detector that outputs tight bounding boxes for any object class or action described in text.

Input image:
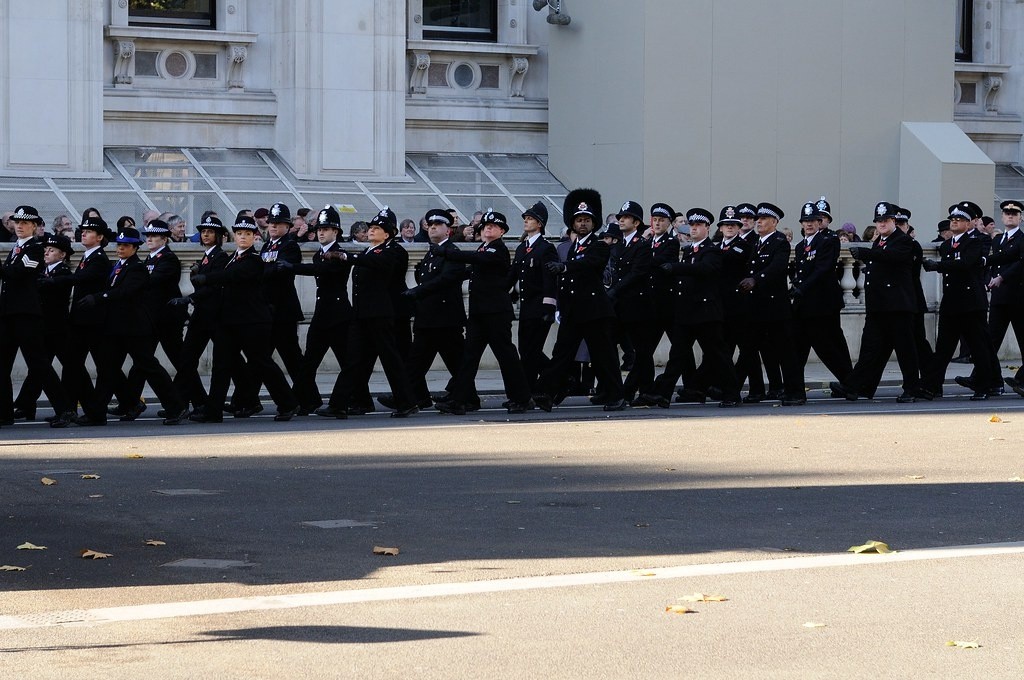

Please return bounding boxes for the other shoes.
[2,350,1022,430]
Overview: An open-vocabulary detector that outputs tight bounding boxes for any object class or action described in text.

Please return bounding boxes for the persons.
[0,188,1024,428]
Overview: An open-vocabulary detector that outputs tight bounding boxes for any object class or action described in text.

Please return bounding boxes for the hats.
[194,202,400,241]
[565,187,835,227]
[8,205,174,254]
[423,201,550,236]
[871,200,1024,221]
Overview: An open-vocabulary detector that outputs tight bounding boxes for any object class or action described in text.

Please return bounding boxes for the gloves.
[400,289,417,299]
[545,262,565,273]
[740,278,755,292]
[849,247,860,259]
[80,295,95,312]
[787,287,802,300]
[276,258,293,275]
[322,250,347,260]
[922,258,938,272]
